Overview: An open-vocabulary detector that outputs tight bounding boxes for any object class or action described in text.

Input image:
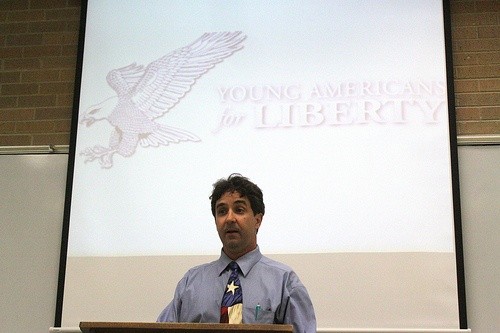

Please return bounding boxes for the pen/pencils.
[256,302,261,321]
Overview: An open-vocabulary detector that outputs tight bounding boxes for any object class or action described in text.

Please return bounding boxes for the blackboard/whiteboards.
[0,142,498,332]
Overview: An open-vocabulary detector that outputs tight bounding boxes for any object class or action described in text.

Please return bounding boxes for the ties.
[219,261,244,324]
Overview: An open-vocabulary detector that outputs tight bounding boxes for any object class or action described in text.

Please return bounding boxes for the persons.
[156,172,317,333]
